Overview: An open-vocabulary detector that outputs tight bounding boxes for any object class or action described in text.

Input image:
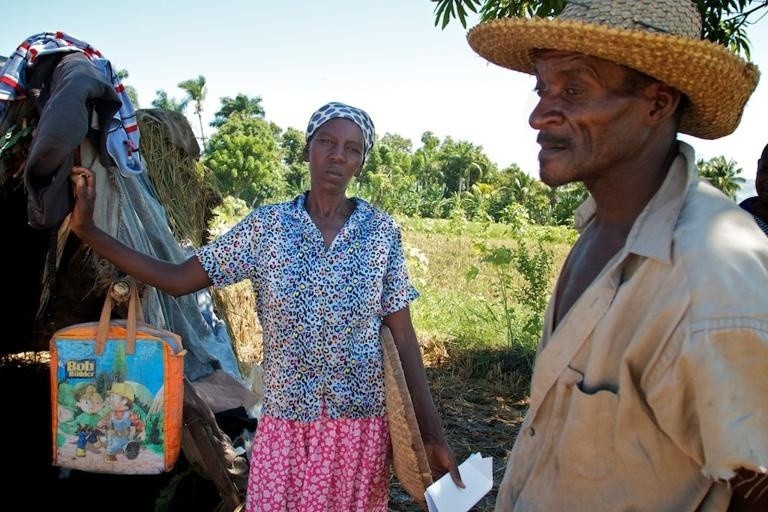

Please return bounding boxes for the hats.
[304,100,378,167]
[463,0,763,141]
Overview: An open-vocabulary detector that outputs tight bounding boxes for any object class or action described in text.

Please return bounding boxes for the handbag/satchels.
[45,274,188,477]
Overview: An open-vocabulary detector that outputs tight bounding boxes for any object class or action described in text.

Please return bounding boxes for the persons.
[726,142,767,234]
[71,101,468,512]
[465,1,766,512]
